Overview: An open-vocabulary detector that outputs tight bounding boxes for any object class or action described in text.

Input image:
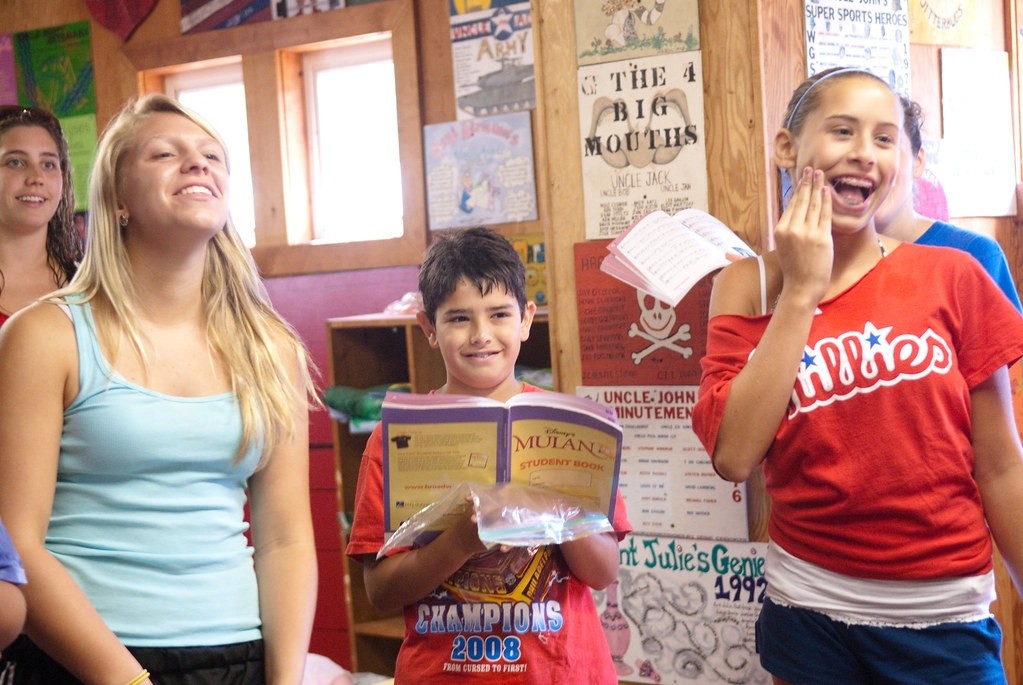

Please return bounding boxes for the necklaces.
[876,239,886,259]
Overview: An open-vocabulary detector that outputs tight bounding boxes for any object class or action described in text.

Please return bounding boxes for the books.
[599,206,762,309]
[381,390,624,534]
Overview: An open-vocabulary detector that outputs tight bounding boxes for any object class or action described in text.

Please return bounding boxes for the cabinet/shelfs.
[324,314,551,677]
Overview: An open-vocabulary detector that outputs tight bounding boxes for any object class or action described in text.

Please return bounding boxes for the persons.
[873,96,1023,316]
[344,228,635,685]
[0,520,29,647]
[0,99,92,331]
[0,93,327,685]
[692,63,1023,685]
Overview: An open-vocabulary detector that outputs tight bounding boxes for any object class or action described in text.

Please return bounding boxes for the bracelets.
[128,669,150,685]
